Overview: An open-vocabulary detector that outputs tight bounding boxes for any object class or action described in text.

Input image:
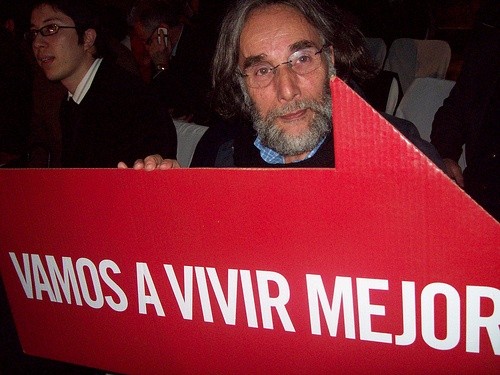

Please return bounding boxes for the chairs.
[172,0,484,173]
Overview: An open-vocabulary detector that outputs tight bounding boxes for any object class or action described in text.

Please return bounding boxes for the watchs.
[155,63,168,72]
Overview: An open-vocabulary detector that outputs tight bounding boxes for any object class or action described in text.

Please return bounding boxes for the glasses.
[23,22,88,42]
[236,40,328,89]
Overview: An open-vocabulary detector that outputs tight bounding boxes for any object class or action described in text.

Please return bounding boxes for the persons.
[0,0,500,222]
[117,0,446,172]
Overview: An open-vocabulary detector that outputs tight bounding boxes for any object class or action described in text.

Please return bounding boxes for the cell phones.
[158,28,168,46]
